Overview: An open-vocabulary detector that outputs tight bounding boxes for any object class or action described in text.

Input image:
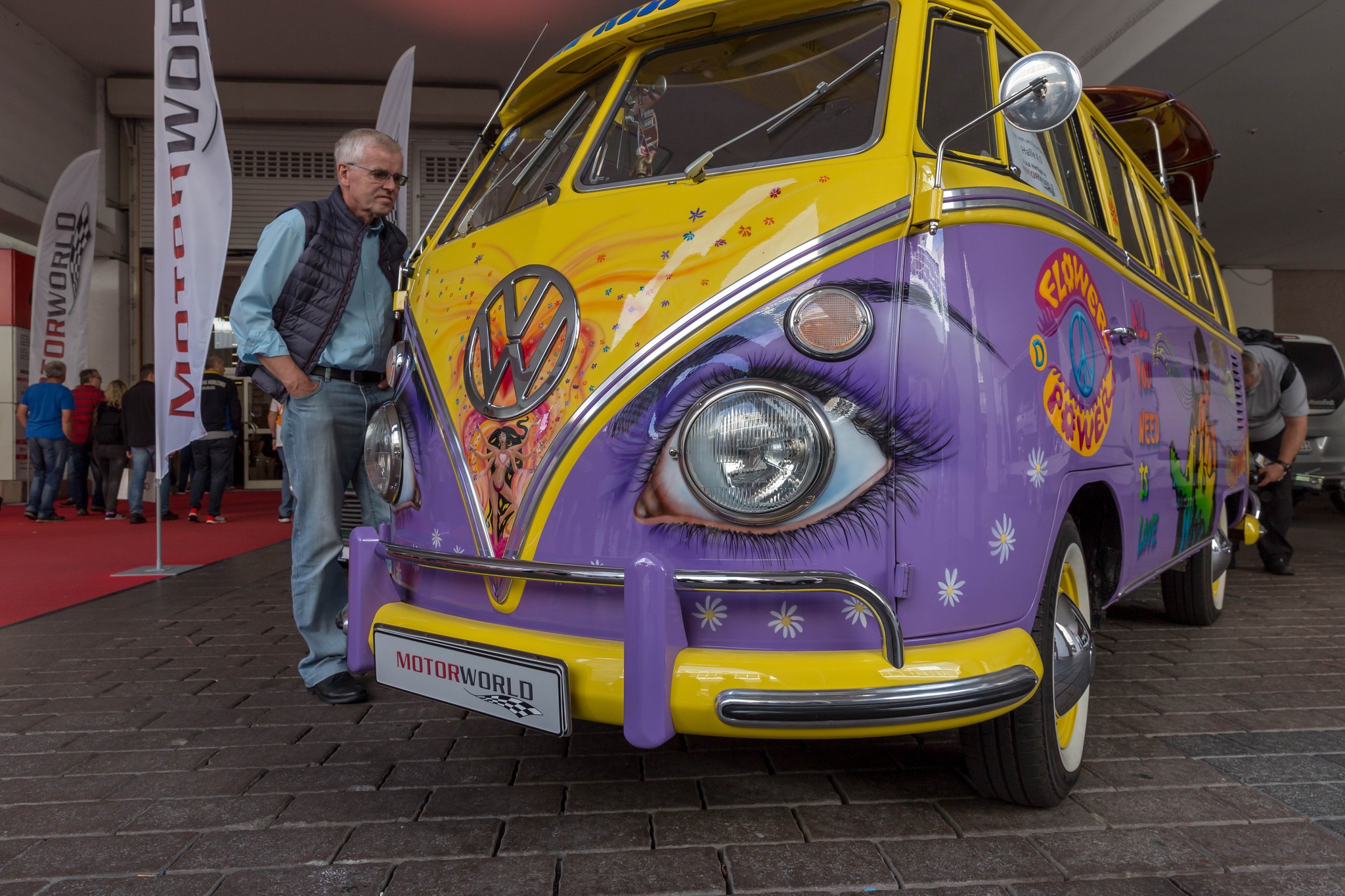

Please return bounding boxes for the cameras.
[1249,474,1262,485]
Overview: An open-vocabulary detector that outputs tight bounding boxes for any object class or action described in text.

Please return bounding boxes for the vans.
[1260,333,1345,514]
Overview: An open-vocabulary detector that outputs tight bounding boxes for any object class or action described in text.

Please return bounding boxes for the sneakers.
[129,514,146,523]
[278,515,290,522]
[23,510,37,520]
[206,515,227,524]
[89,505,106,513]
[105,511,128,520]
[77,508,88,515]
[58,497,76,507]
[162,513,178,520]
[188,508,199,521]
[36,513,66,522]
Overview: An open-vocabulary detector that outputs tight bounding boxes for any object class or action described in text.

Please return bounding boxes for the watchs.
[1275,459,1292,472]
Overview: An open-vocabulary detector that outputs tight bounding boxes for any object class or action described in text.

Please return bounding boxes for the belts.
[309,365,387,385]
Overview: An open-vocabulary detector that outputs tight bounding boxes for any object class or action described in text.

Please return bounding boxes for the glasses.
[347,163,409,186]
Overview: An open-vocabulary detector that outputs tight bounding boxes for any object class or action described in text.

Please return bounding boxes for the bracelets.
[272,438,276,439]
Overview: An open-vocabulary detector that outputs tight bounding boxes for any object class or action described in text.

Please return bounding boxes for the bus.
[344,0,1249,807]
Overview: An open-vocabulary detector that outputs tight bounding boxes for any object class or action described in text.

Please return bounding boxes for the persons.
[18,355,296,525]
[229,129,413,705]
[1226,345,1309,575]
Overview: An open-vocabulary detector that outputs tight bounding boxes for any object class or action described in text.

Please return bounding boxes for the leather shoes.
[1264,561,1295,576]
[306,672,365,703]
